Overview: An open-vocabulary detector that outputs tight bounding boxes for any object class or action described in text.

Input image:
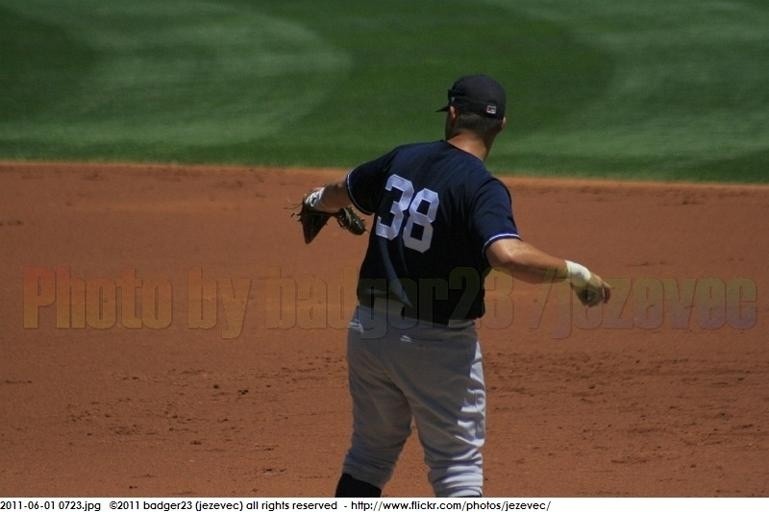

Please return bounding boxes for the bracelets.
[564,259,592,288]
[304,187,339,213]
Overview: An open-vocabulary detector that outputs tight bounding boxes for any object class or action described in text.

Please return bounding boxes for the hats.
[435,74,506,121]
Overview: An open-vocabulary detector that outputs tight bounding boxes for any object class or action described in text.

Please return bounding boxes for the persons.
[305,74,611,497]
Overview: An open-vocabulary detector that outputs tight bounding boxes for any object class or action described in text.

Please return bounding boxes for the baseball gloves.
[284,185,371,244]
